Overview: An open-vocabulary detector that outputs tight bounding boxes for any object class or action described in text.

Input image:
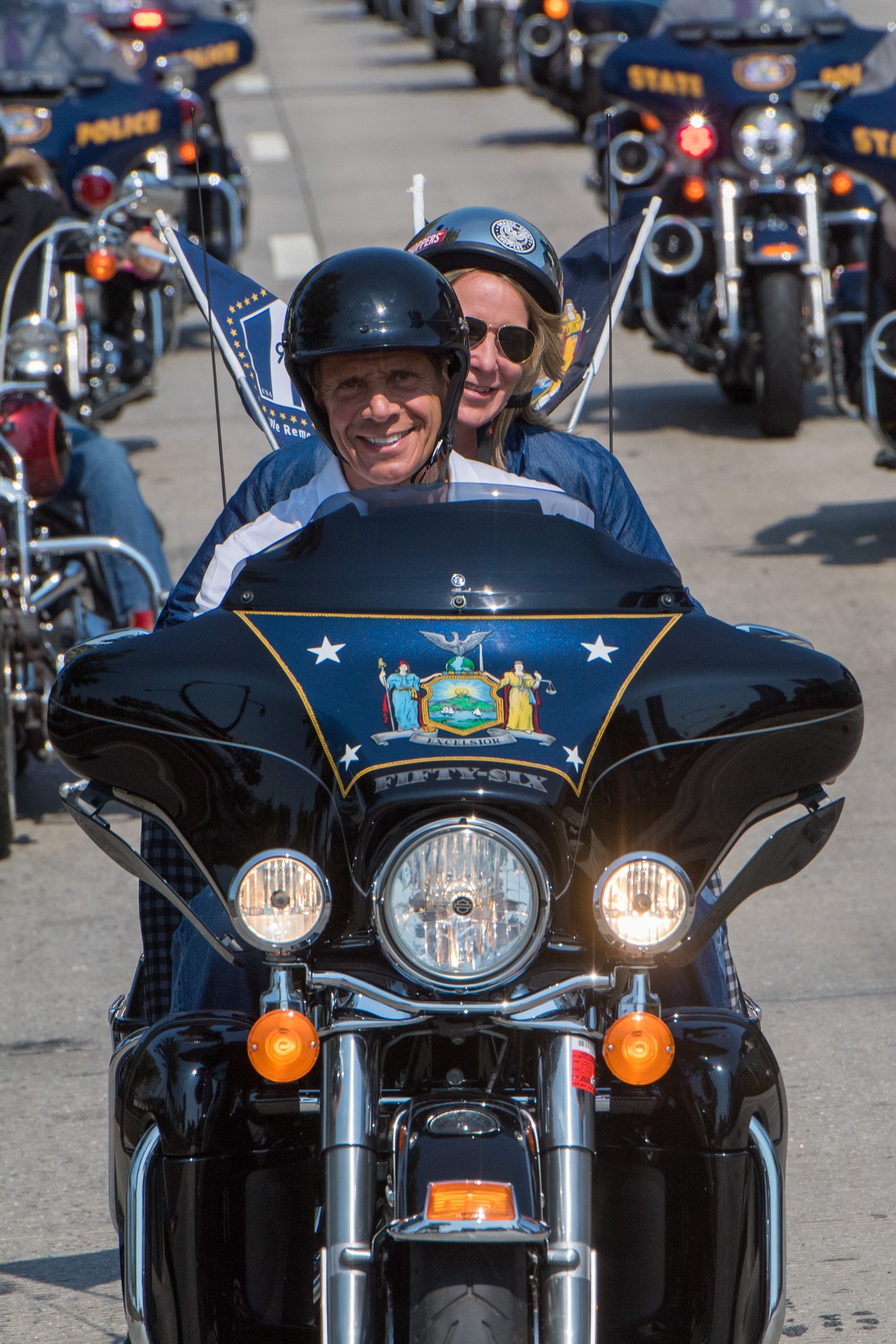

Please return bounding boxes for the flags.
[162,224,325,451]
[529,210,653,418]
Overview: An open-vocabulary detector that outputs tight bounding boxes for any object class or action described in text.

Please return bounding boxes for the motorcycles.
[362,0,896,472]
[42,472,865,1343]
[0,1,265,850]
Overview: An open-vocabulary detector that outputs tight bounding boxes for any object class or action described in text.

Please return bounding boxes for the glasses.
[463,315,537,364]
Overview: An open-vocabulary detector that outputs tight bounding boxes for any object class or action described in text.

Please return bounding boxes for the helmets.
[404,207,564,322]
[282,246,469,391]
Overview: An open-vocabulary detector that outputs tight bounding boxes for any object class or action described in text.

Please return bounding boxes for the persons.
[1,0,896,1017]
